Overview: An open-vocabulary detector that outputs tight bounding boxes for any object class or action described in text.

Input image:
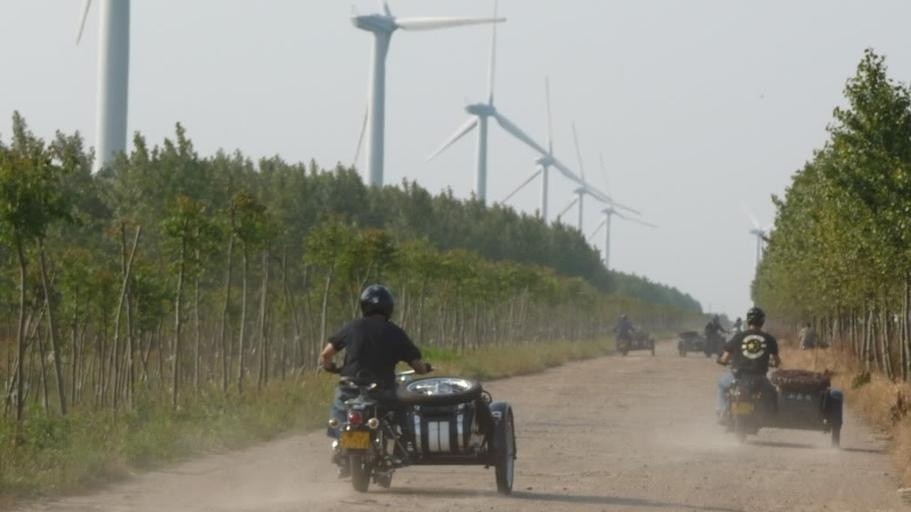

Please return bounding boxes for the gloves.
[326,363,342,372]
[424,362,432,372]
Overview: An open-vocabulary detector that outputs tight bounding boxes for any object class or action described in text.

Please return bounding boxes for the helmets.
[360,284,393,317]
[746,306,765,326]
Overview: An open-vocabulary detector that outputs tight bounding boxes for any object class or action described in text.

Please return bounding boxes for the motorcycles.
[616,331,843,448]
[324,367,517,494]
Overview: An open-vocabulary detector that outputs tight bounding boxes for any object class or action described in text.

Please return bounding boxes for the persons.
[798,322,817,348]
[320,284,432,437]
[704,315,743,336]
[613,314,636,350]
[717,307,781,424]
